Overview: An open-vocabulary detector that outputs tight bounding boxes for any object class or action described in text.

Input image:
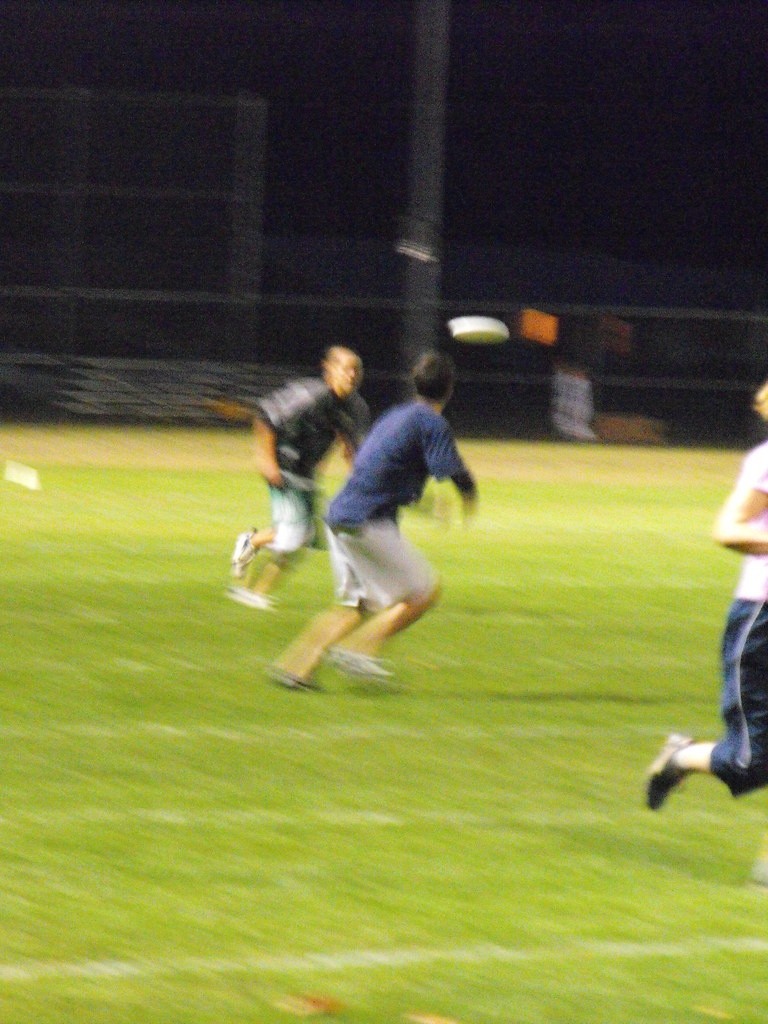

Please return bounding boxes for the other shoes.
[267,665,322,691]
[231,586,273,613]
[328,648,394,680]
[232,528,257,579]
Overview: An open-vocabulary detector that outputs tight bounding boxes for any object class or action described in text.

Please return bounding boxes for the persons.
[229,345,372,606]
[272,354,478,688]
[556,363,594,440]
[644,383,768,808]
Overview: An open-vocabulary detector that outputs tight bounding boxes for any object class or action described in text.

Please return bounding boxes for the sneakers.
[647,735,696,809]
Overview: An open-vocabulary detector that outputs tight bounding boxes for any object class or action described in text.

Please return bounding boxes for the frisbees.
[447,313,511,347]
